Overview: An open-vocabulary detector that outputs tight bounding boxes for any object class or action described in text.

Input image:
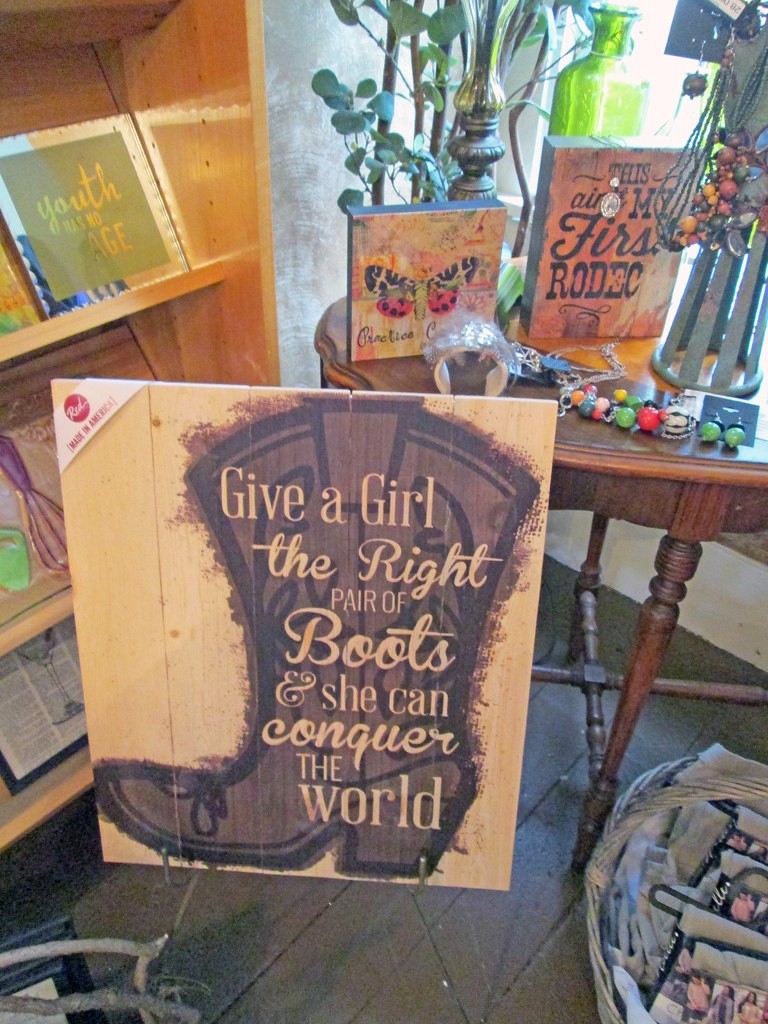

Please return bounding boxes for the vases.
[550,3,640,137]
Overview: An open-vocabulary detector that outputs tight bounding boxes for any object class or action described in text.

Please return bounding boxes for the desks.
[311,288,766,869]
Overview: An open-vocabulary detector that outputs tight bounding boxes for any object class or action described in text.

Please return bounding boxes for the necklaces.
[545,338,625,417]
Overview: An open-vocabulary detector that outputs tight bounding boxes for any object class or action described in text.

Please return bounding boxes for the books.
[1,614,90,797]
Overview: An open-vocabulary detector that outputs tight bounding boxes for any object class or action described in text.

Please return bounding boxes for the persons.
[729,889,755,922]
[670,946,759,1024]
[726,833,768,863]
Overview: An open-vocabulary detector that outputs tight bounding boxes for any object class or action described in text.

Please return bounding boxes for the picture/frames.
[0,914,109,1023]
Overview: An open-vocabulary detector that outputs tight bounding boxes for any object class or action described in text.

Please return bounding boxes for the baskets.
[583,755,768,1024]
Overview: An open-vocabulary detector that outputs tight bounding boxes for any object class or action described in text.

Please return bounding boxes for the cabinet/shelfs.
[0,0,284,856]
